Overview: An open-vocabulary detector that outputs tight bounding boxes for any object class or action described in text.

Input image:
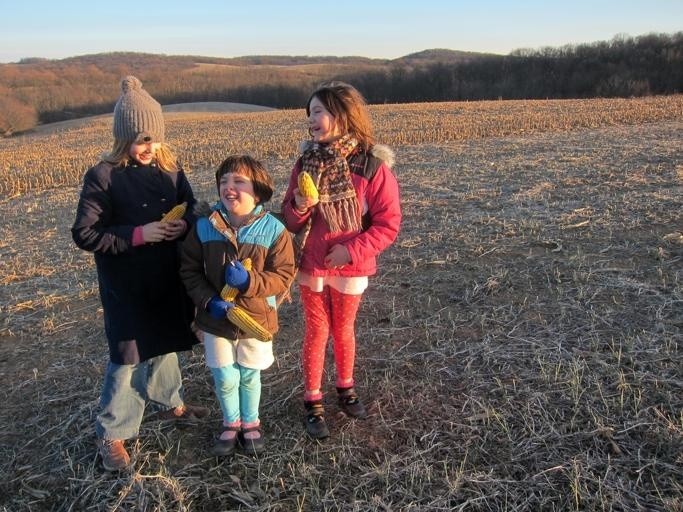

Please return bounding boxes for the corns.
[160,202,187,223]
[220,258,251,301]
[226,308,272,342]
[297,171,319,199]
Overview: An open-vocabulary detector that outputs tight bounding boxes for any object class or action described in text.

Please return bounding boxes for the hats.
[114,75,165,142]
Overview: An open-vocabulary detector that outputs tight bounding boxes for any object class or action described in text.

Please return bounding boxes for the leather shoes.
[338,393,366,419]
[242,424,265,453]
[304,405,328,439]
[214,427,239,456]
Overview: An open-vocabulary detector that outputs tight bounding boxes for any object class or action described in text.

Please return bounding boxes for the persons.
[69,76,199,472]
[276,81,402,440]
[179,155,297,456]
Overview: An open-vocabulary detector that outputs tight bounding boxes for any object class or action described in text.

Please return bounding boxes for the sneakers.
[164,406,209,420]
[97,439,129,470]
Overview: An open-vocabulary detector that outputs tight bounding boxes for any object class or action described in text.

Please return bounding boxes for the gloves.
[208,298,233,319]
[225,262,249,289]
